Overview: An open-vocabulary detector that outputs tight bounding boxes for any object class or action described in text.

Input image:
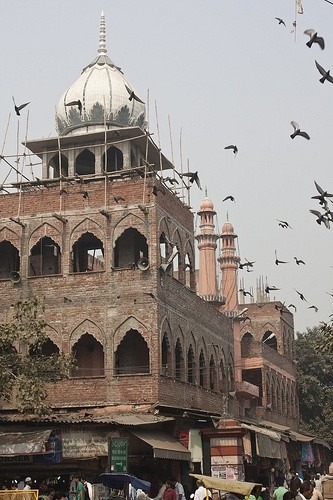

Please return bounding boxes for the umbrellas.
[98,473,151,491]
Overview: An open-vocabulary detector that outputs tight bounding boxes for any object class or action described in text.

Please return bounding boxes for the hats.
[25,477,31,482]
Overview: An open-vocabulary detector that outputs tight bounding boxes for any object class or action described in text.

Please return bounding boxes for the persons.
[129,480,213,500]
[2,474,94,500]
[257,472,330,500]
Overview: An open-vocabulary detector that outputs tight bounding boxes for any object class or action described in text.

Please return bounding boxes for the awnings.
[132,430,191,460]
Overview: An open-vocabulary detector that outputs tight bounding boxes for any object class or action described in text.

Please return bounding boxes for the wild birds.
[178,171,203,192]
[222,195,236,204]
[223,145,238,158]
[10,95,31,116]
[125,85,146,105]
[64,99,83,117]
[236,218,333,319]
[309,179,333,232]
[272,1,332,87]
[289,121,310,141]
